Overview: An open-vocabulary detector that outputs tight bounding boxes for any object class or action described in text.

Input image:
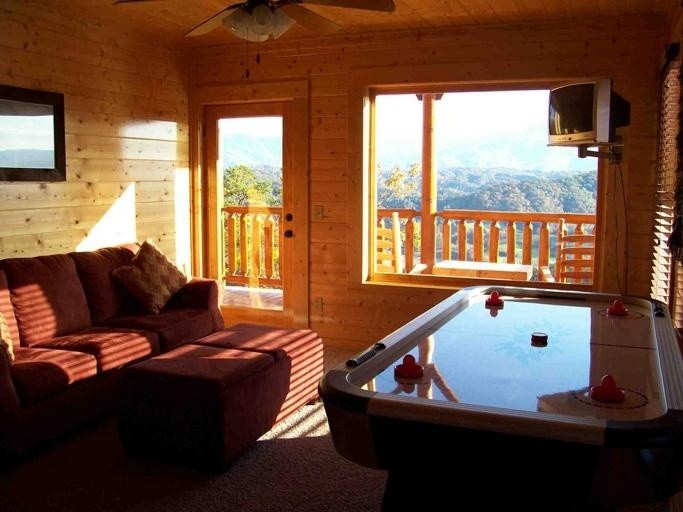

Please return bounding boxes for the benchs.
[121,323,322,469]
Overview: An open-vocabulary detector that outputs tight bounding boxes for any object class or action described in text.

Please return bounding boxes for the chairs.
[376,212,427,275]
[540,218,596,284]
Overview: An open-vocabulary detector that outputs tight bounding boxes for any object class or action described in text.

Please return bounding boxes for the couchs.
[0,248,226,462]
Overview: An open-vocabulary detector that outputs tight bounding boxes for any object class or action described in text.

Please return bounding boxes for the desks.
[320,285,682,511]
[432,260,533,281]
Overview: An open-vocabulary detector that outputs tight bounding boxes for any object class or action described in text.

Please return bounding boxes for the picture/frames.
[0,83,66,179]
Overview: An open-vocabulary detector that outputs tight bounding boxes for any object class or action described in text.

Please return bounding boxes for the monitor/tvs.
[546,77,631,146]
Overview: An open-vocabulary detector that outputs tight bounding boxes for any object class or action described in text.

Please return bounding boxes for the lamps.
[222,5,295,44]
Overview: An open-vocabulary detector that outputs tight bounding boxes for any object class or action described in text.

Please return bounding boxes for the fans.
[109,1,395,38]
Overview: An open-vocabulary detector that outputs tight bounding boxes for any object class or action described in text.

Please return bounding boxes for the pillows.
[109,243,187,314]
